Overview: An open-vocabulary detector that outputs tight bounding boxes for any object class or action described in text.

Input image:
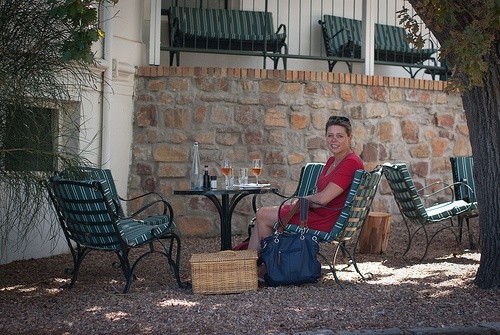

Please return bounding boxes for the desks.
[174,187,279,251]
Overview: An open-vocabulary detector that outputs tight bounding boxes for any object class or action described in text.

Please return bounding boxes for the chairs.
[44,168,182,293]
[248,164,325,240]
[274,166,384,291]
[450,156,476,249]
[381,163,477,264]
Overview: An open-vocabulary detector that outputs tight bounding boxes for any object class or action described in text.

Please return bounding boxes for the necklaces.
[333,149,351,167]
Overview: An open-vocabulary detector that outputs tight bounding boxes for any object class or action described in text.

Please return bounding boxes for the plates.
[238,182,270,188]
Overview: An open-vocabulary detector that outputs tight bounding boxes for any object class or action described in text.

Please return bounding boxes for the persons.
[248,116,365,255]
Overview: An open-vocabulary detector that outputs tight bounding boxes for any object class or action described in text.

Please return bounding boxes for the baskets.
[188,249,258,295]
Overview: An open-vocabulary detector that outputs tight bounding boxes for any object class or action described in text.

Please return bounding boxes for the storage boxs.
[189,252,258,295]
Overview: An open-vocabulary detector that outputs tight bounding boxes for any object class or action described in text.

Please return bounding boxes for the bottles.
[210,175,218,188]
[190,141,201,190]
[203,165,210,189]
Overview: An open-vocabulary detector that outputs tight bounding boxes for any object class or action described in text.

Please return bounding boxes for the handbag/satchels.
[260,197,321,287]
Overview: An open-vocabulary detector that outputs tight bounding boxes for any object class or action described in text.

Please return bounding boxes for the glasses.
[329,116,349,122]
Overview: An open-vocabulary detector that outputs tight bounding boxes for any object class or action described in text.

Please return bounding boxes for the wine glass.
[219,158,234,189]
[252,159,263,185]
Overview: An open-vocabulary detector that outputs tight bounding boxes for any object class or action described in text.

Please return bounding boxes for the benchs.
[168,7,287,70]
[318,15,440,80]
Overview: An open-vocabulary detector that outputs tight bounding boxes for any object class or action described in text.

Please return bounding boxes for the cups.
[226,169,234,188]
[238,168,249,186]
